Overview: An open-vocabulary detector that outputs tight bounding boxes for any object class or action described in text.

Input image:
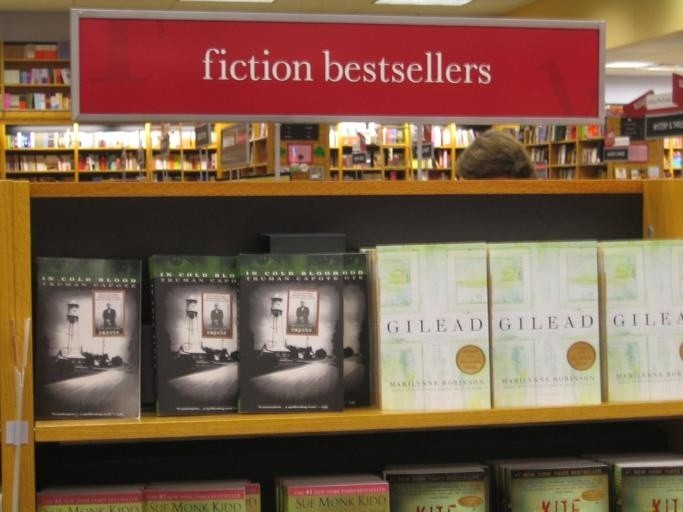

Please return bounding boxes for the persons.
[453,132,535,181]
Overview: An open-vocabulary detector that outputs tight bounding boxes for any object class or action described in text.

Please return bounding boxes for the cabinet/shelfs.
[1,178,683,511]
[2,41,680,180]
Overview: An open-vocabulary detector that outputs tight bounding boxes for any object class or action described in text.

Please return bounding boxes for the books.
[78,131,146,183]
[4,44,75,182]
[281,125,475,182]
[32,237,683,421]
[504,124,683,179]
[32,451,683,511]
[154,122,267,182]
